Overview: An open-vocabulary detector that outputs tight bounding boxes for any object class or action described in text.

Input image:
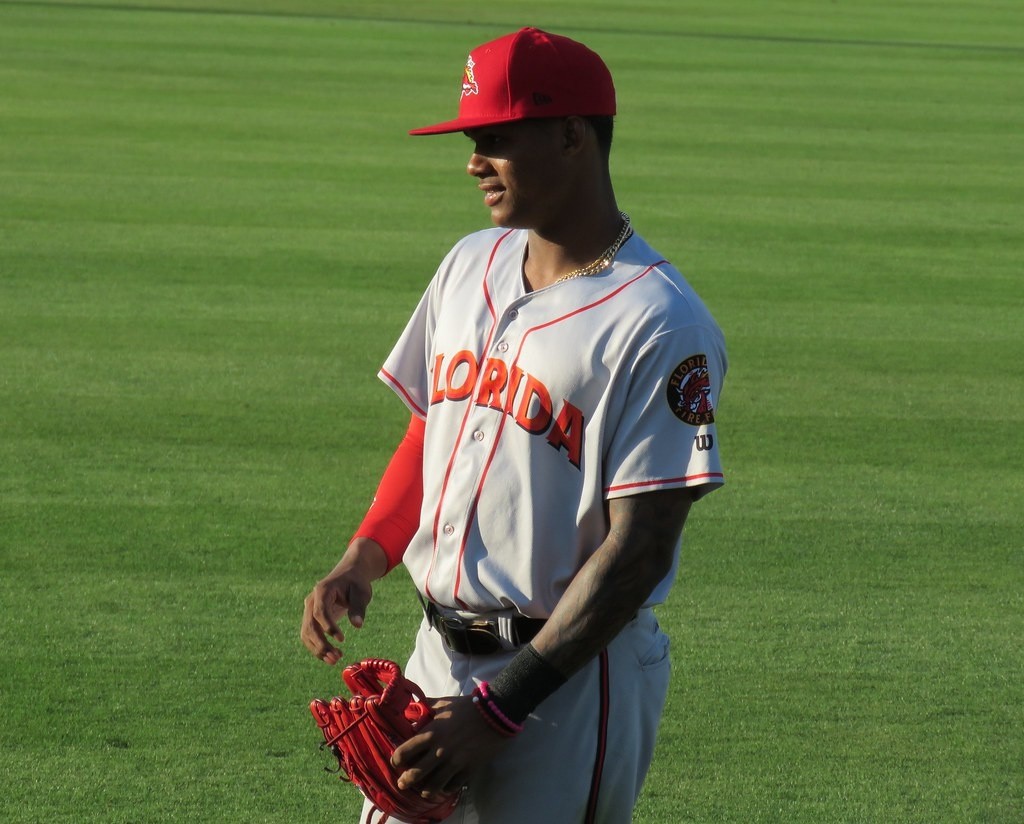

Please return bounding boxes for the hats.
[407,27,616,135]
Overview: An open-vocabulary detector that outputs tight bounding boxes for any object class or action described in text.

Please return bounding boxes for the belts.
[416,587,636,656]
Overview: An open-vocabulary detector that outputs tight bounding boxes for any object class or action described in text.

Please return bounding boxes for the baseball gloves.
[309,654,469,824]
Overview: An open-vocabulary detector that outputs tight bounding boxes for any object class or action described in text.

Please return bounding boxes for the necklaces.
[556,210,632,282]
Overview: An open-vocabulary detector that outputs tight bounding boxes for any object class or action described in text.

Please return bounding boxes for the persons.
[300,26,729,824]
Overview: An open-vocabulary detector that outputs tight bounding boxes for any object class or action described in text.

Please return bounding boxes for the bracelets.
[473,682,524,737]
